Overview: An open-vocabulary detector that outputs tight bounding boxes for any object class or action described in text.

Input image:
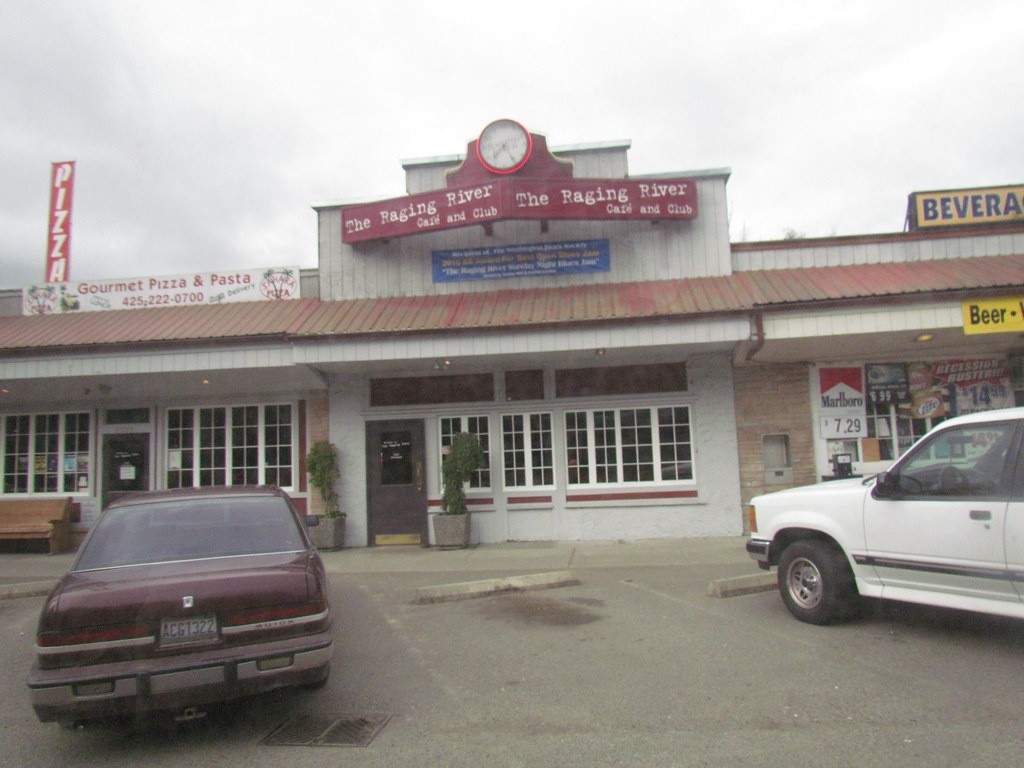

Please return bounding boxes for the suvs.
[746,405,1024,628]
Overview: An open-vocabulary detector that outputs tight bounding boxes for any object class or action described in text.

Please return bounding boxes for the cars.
[28,485,334,732]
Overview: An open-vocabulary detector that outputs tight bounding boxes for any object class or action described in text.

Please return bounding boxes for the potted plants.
[305,439,346,552]
[431,432,485,549]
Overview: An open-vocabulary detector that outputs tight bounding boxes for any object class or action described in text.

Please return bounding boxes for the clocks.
[475,118,533,175]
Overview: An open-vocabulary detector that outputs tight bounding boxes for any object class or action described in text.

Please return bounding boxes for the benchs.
[0,497,73,556]
